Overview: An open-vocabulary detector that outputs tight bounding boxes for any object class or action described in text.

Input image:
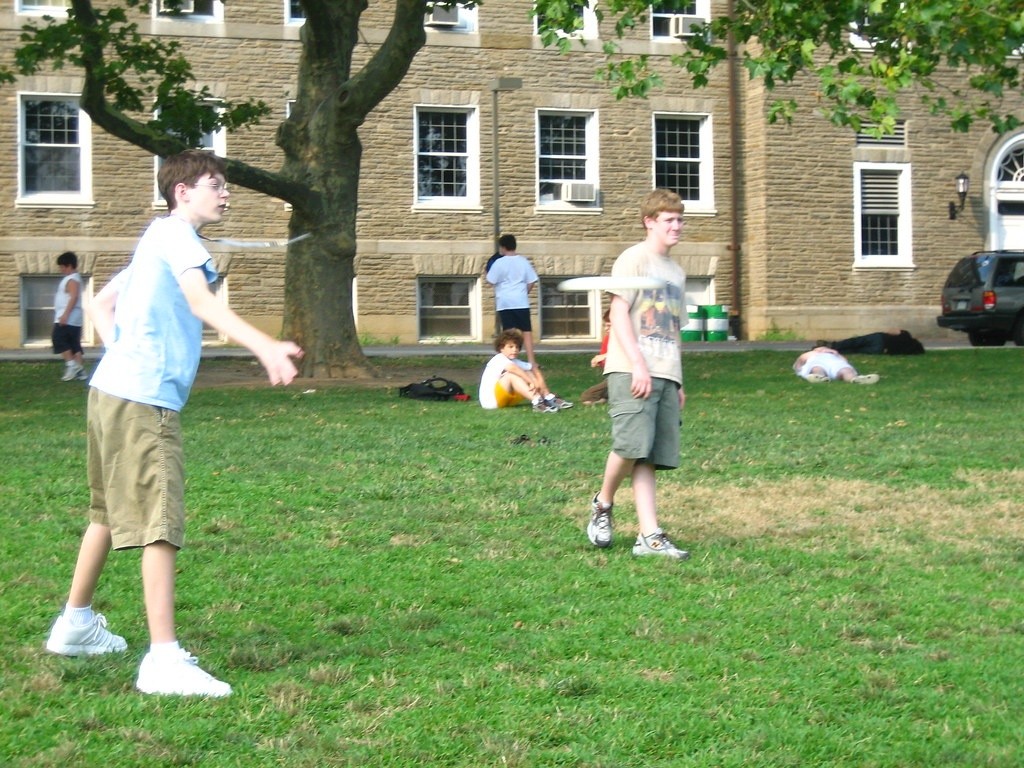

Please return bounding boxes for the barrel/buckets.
[681,305,702,341]
[705,305,729,342]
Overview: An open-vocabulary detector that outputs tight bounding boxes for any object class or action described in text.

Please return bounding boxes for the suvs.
[936,247,1024,346]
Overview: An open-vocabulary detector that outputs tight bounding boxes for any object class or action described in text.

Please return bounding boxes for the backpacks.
[399,377,464,402]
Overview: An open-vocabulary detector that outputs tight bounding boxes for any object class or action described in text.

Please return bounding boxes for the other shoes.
[59,360,88,382]
[805,374,831,383]
[851,373,881,385]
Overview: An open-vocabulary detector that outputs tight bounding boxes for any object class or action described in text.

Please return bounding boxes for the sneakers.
[134,645,233,698]
[532,396,574,414]
[587,492,613,547]
[632,530,689,561]
[46,616,128,655]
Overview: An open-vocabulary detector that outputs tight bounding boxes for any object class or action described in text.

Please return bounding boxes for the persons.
[790,346,881,385]
[586,188,689,560]
[46,149,306,696]
[50,250,88,383]
[480,329,575,414]
[582,307,612,405]
[485,233,541,366]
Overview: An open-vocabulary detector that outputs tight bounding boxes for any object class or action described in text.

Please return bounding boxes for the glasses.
[192,182,228,192]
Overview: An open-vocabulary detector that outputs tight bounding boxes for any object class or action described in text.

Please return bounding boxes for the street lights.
[490,76,524,341]
